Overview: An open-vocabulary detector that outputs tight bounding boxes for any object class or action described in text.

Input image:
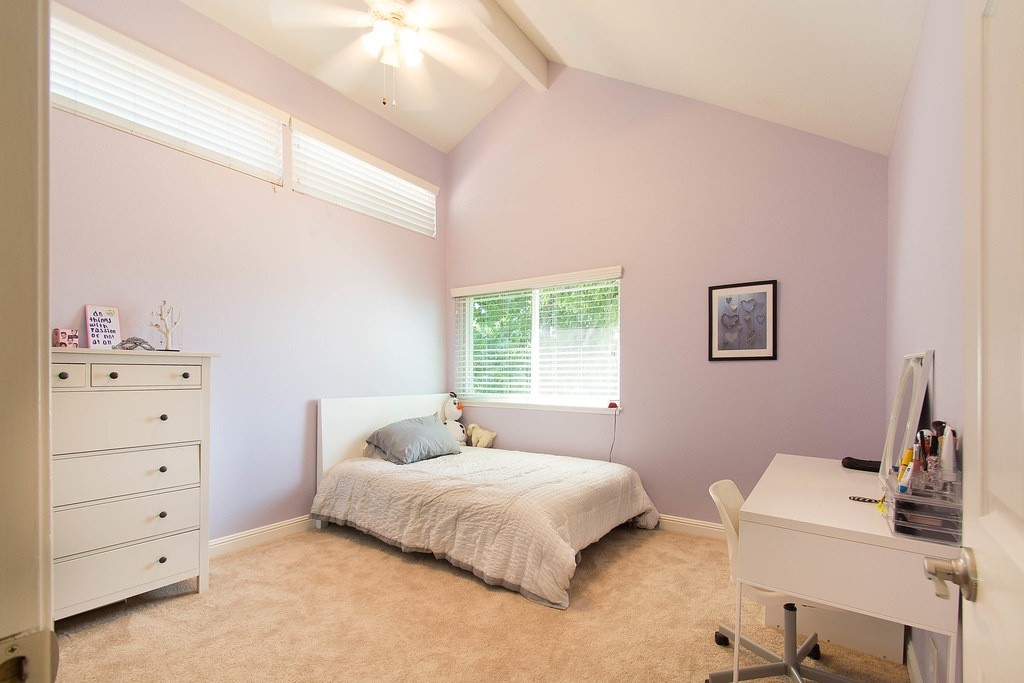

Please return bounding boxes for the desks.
[734,453,962,683]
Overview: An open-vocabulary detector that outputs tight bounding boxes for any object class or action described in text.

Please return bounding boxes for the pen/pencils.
[920,430,929,471]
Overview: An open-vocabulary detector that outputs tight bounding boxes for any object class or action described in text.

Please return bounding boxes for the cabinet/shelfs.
[52,346,221,623]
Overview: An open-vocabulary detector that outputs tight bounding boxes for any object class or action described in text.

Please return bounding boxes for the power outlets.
[929,636,939,683]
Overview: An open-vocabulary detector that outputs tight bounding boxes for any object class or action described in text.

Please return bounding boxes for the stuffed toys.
[466,424,497,449]
[444,392,468,445]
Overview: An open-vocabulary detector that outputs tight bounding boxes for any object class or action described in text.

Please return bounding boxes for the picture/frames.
[708,279,776,361]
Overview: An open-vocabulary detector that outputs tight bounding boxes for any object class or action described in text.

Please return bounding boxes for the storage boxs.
[53,328,78,349]
[886,470,962,546]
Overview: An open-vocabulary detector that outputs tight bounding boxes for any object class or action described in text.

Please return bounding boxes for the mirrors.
[878,350,934,487]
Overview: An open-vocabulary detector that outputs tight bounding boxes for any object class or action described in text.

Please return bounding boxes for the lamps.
[363,13,426,107]
[607,399,619,409]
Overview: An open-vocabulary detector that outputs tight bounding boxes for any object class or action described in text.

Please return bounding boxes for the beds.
[309,393,660,611]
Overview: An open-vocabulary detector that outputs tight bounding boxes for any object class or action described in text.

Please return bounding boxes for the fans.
[268,0,498,92]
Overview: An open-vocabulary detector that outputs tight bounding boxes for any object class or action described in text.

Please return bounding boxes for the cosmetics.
[892,420,957,494]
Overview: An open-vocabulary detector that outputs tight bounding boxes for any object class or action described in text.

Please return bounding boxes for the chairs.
[705,478,865,682]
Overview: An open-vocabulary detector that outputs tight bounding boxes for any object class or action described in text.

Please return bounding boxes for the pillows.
[363,412,462,465]
